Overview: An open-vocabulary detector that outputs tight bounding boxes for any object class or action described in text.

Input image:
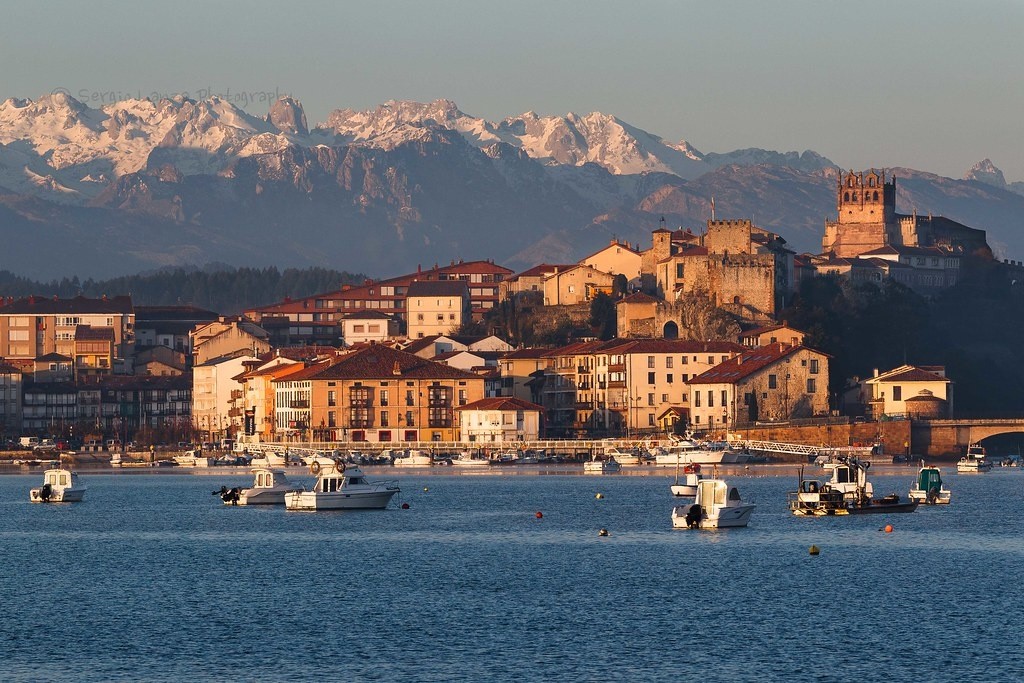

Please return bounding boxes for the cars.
[33,442,57,452]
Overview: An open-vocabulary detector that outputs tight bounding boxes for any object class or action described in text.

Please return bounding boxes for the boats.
[29,468,86,502]
[175,450,214,467]
[394,443,565,465]
[212,466,306,505]
[668,479,757,528]
[957,443,992,472]
[907,465,952,504]
[581,446,754,471]
[671,468,705,496]
[785,452,921,517]
[284,458,402,509]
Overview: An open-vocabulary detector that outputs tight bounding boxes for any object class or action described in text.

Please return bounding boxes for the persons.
[807,482,815,494]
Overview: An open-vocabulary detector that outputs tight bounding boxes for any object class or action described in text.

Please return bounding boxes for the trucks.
[17,436,39,449]
